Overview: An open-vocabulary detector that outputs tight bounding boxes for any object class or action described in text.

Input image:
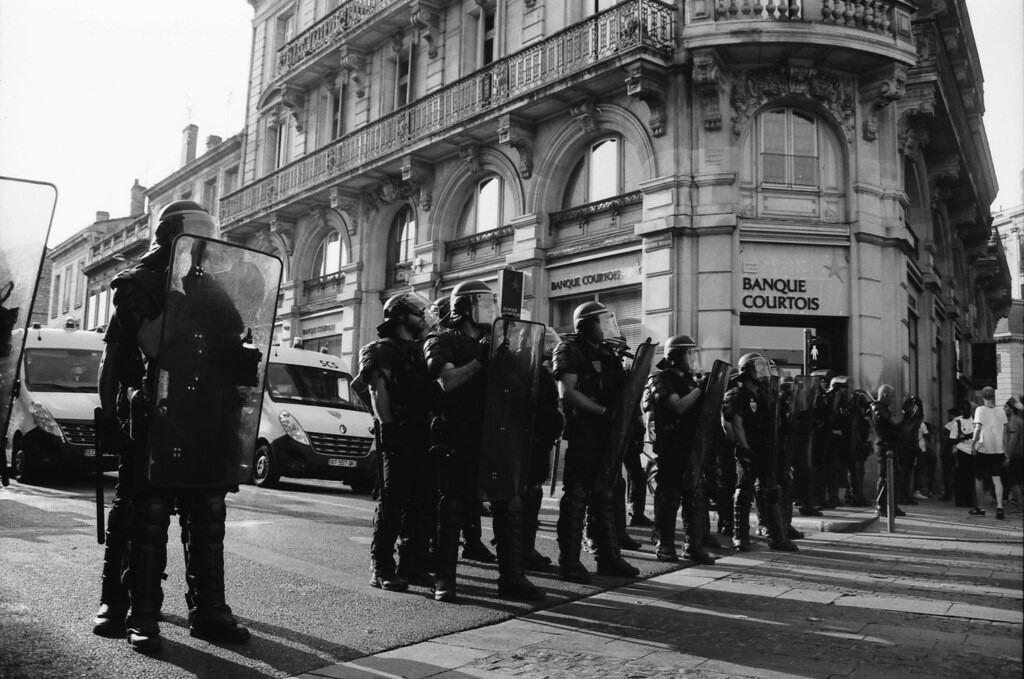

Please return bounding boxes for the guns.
[368,417,406,522]
[93,401,138,544]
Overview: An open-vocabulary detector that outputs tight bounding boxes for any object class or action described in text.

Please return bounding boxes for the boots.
[93,597,251,653]
[559,510,721,576]
[798,490,919,517]
[716,522,804,552]
[369,537,552,604]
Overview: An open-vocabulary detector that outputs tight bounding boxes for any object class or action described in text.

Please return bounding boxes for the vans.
[238,336,380,494]
[0,318,120,483]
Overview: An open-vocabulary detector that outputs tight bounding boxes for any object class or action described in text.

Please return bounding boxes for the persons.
[108,202,262,652]
[552,301,640,581]
[352,280,933,599]
[91,315,194,640]
[967,386,1009,521]
[421,280,546,600]
[358,292,435,591]
[1001,397,1024,515]
[942,387,977,520]
[643,334,717,568]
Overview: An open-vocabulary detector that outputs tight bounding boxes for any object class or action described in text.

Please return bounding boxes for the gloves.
[476,334,492,365]
[602,406,617,420]
[695,372,710,392]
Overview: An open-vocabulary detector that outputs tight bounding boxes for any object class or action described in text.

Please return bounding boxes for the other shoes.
[968,497,1018,520]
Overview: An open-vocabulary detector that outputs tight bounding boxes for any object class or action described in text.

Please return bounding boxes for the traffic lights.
[809,340,830,366]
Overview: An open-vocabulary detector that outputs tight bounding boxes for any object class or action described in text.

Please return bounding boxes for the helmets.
[664,335,703,373]
[154,200,220,251]
[768,359,780,377]
[430,295,450,323]
[376,290,439,338]
[516,326,562,357]
[606,331,627,350]
[737,352,771,380]
[779,369,851,398]
[450,280,496,329]
[573,301,621,341]
